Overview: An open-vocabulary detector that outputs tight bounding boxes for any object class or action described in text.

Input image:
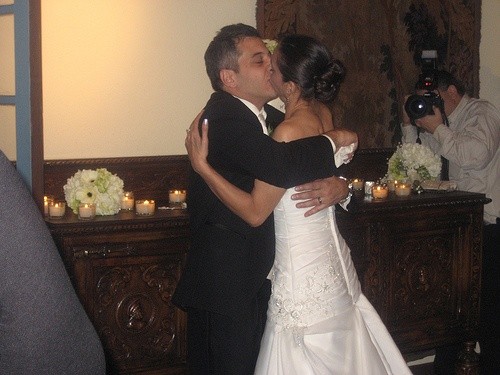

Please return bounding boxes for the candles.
[169,190,186,203]
[373,186,387,198]
[352,179,363,189]
[396,184,410,196]
[43,191,155,221]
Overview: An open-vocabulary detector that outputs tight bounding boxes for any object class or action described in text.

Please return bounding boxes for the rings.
[186,127,190,132]
[318,197,322,205]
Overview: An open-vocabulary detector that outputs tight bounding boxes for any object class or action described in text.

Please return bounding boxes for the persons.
[0,148,108,375]
[396,66,500,375]
[179,24,415,375]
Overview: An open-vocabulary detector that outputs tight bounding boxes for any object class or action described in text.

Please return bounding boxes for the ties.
[258,114,269,137]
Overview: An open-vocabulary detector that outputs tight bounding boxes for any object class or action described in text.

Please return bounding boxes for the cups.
[44,195,55,217]
[48,201,66,220]
[121,191,134,212]
[351,178,364,190]
[371,184,389,199]
[168,190,186,208]
[135,199,156,216]
[77,203,96,220]
[395,183,411,197]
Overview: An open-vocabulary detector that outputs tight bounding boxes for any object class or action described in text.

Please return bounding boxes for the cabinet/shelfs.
[44,189,492,375]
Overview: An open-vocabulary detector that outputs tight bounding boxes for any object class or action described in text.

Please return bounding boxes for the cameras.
[405,93,445,120]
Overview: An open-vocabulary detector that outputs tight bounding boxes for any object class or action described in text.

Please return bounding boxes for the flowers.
[377,142,442,194]
[63,168,125,216]
[262,39,278,55]
